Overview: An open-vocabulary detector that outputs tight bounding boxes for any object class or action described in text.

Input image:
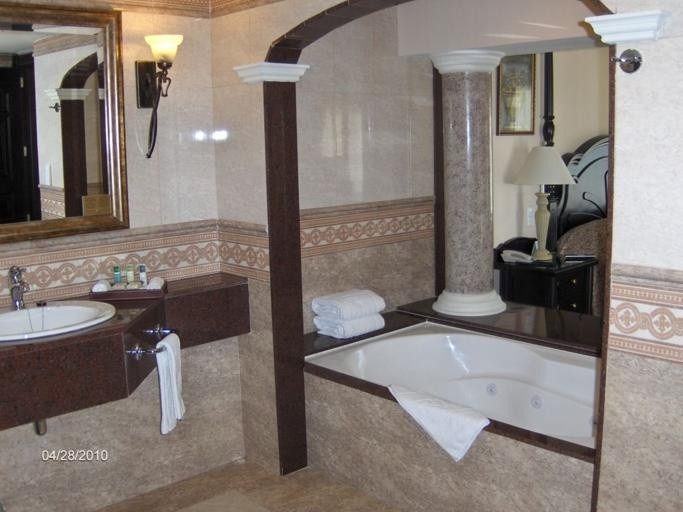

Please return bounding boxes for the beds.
[542,50,610,320]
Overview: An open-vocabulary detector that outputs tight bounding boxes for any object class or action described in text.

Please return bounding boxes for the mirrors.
[0,0,131,246]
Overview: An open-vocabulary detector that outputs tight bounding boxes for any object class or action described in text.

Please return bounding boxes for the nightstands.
[499,255,600,315]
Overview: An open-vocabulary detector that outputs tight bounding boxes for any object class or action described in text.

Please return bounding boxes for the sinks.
[0,298,117,342]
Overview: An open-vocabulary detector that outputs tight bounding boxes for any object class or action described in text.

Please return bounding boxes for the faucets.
[9,266,30,307]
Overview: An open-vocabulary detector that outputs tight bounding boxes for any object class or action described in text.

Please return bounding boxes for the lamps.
[133,30,184,162]
[510,145,576,265]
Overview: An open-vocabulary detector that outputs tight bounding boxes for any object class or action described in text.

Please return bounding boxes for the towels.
[311,285,386,321]
[312,314,385,342]
[387,382,493,464]
[154,329,188,438]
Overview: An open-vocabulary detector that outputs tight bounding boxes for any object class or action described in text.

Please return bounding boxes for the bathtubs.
[301,320,601,450]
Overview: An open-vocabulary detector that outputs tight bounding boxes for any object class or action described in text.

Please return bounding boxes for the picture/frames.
[494,52,537,138]
